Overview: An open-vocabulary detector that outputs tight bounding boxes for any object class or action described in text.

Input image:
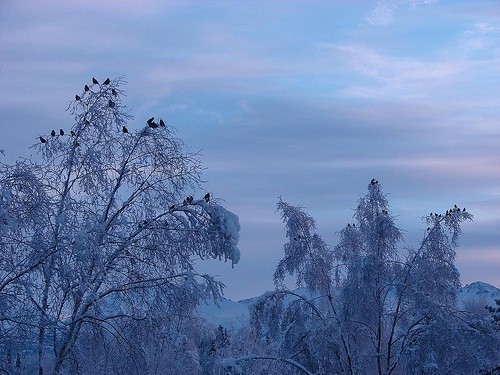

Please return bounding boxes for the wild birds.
[39,129,80,147]
[122,125,129,132]
[138,192,211,229]
[382,210,389,215]
[348,223,356,228]
[147,117,165,128]
[293,235,301,241]
[426,203,466,231]
[371,178,379,184]
[74,75,119,108]
